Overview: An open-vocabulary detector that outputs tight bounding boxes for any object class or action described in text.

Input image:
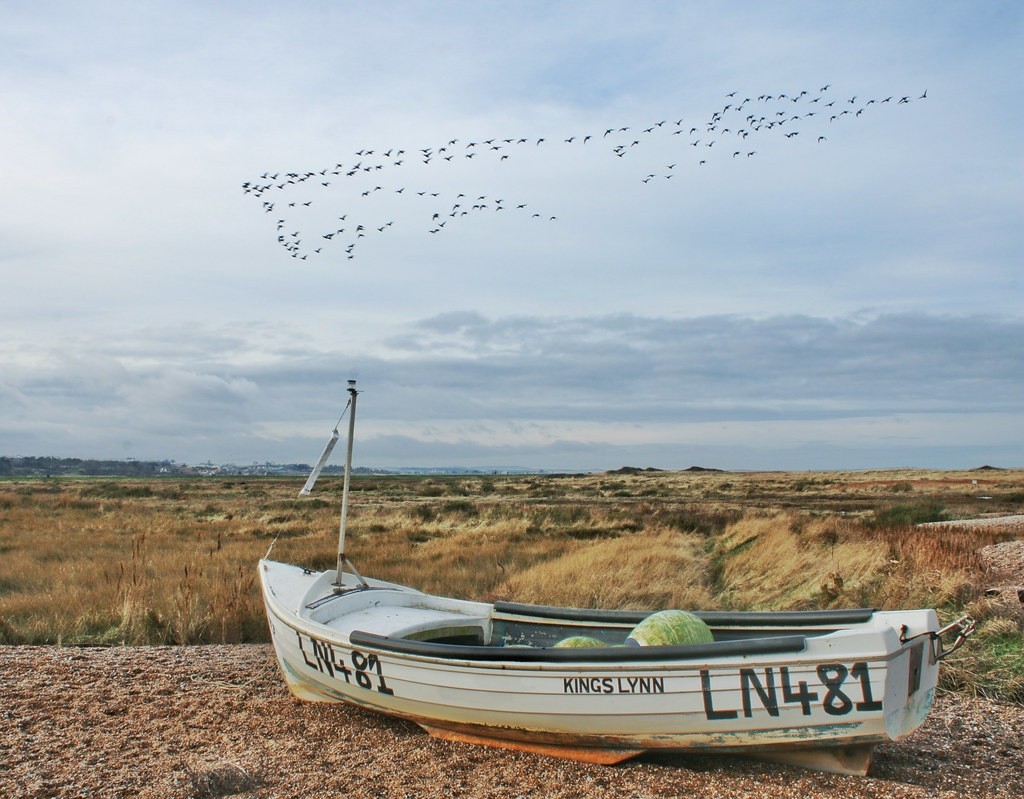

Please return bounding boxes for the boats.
[255,381,973,766]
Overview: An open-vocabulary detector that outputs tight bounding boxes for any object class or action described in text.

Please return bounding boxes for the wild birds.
[243,83,927,265]
